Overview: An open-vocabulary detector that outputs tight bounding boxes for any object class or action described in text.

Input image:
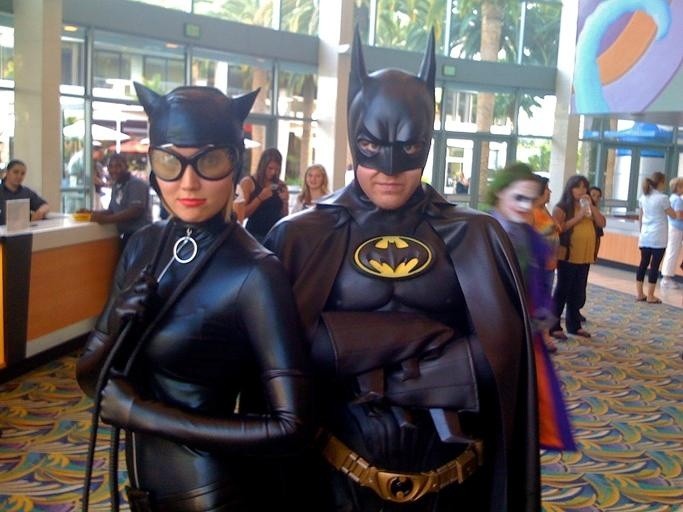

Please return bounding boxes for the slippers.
[647,297,662,304]
[638,296,648,300]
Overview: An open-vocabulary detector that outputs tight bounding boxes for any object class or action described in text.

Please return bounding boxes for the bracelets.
[257,196,264,202]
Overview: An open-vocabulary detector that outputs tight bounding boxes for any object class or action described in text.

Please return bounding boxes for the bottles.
[579,198,592,218]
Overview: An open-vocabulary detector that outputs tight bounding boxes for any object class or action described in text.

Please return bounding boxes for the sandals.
[550,329,567,339]
[573,329,590,337]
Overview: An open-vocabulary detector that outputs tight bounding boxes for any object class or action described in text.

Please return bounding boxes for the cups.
[580,198,592,219]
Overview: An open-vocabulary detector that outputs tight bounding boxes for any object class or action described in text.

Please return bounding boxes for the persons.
[549,174,597,340]
[659,177,683,288]
[485,160,577,454]
[638,172,676,303]
[234,147,288,241]
[0,143,5,170]
[344,165,356,186]
[0,160,48,225]
[86,143,114,210]
[290,164,331,212]
[532,173,561,352]
[75,155,151,245]
[239,22,541,511]
[130,154,149,184]
[580,186,606,322]
[67,137,90,196]
[455,172,468,194]
[75,81,301,512]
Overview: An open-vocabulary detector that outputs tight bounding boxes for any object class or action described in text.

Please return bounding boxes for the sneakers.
[661,282,678,289]
[661,279,679,286]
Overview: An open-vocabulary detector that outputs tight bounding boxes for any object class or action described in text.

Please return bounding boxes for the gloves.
[75,265,160,387]
[324,311,460,377]
[356,338,479,415]
[98,371,307,446]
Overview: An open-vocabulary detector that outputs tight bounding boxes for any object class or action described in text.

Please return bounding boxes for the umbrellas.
[107,141,151,155]
[63,122,130,141]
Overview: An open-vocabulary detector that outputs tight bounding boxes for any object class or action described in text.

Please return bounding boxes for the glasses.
[147,144,238,181]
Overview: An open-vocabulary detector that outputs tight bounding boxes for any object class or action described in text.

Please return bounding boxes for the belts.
[124,479,241,511]
[317,430,486,503]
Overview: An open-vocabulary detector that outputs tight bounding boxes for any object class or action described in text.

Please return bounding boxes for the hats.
[134,82,261,186]
[485,162,548,208]
[347,26,436,172]
